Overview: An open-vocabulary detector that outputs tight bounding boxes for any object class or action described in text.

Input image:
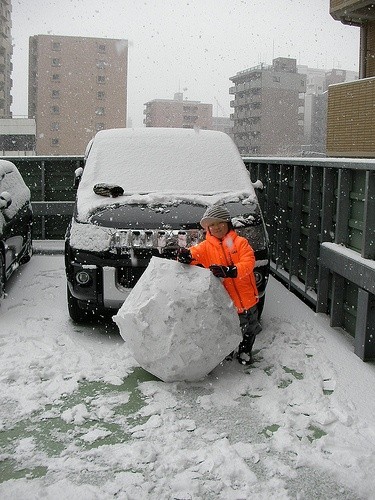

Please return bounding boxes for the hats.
[199,205,230,228]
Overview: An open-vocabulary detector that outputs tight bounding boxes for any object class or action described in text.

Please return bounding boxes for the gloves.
[209,264,237,278]
[178,248,191,263]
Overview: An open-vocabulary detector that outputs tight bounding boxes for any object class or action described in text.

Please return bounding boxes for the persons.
[178,204,258,364]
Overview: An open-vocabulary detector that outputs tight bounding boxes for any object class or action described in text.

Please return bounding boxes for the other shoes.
[239,349,251,364]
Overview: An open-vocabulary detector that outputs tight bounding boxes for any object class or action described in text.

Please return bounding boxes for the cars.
[0,159,34,300]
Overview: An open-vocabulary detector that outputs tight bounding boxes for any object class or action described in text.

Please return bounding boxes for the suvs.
[63,126,272,324]
